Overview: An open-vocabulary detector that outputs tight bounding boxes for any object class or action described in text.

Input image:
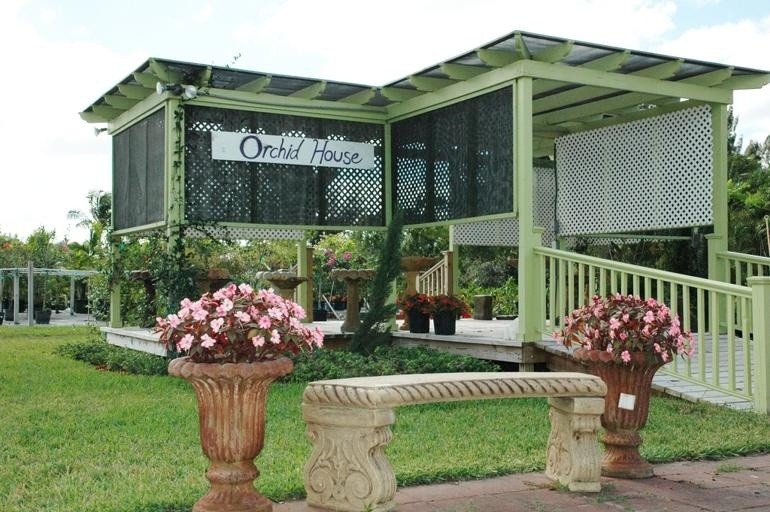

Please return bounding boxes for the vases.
[328,269,374,332]
[574,349,673,479]
[168,357,293,512]
[408,312,429,333]
[434,311,456,334]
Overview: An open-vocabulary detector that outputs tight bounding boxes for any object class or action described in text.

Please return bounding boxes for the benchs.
[302,371,607,512]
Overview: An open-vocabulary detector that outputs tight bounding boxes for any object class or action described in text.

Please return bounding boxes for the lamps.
[156,81,178,96]
[178,84,197,99]
[95,127,107,135]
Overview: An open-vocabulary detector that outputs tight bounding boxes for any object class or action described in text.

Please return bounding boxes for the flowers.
[154,284,323,362]
[430,294,465,314]
[325,246,367,268]
[553,293,694,365]
[397,294,432,315]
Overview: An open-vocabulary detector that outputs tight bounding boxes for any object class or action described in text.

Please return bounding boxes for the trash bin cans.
[473,295,492,319]
